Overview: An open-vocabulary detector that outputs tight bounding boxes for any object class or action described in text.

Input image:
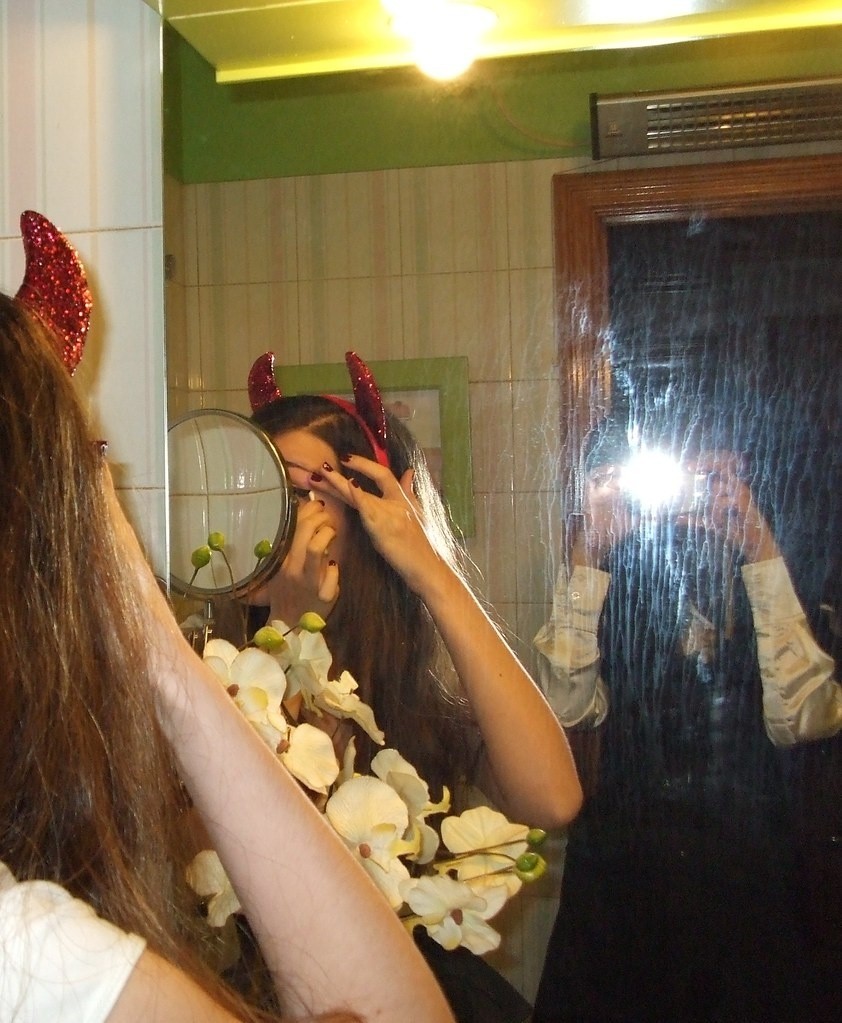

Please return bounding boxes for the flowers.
[179,535,549,956]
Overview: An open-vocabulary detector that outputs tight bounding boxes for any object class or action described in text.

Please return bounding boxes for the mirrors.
[165,1,842,1022]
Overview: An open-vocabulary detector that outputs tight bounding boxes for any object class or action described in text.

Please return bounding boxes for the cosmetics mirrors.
[167,409,291,641]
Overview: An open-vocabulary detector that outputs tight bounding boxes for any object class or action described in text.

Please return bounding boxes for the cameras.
[626,465,710,516]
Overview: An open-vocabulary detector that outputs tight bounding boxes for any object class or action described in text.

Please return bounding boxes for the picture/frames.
[270,355,478,541]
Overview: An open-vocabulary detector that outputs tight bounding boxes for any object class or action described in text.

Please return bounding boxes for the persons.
[1,209,458,1021]
[534,412,842,1023]
[206,349,583,1022]
